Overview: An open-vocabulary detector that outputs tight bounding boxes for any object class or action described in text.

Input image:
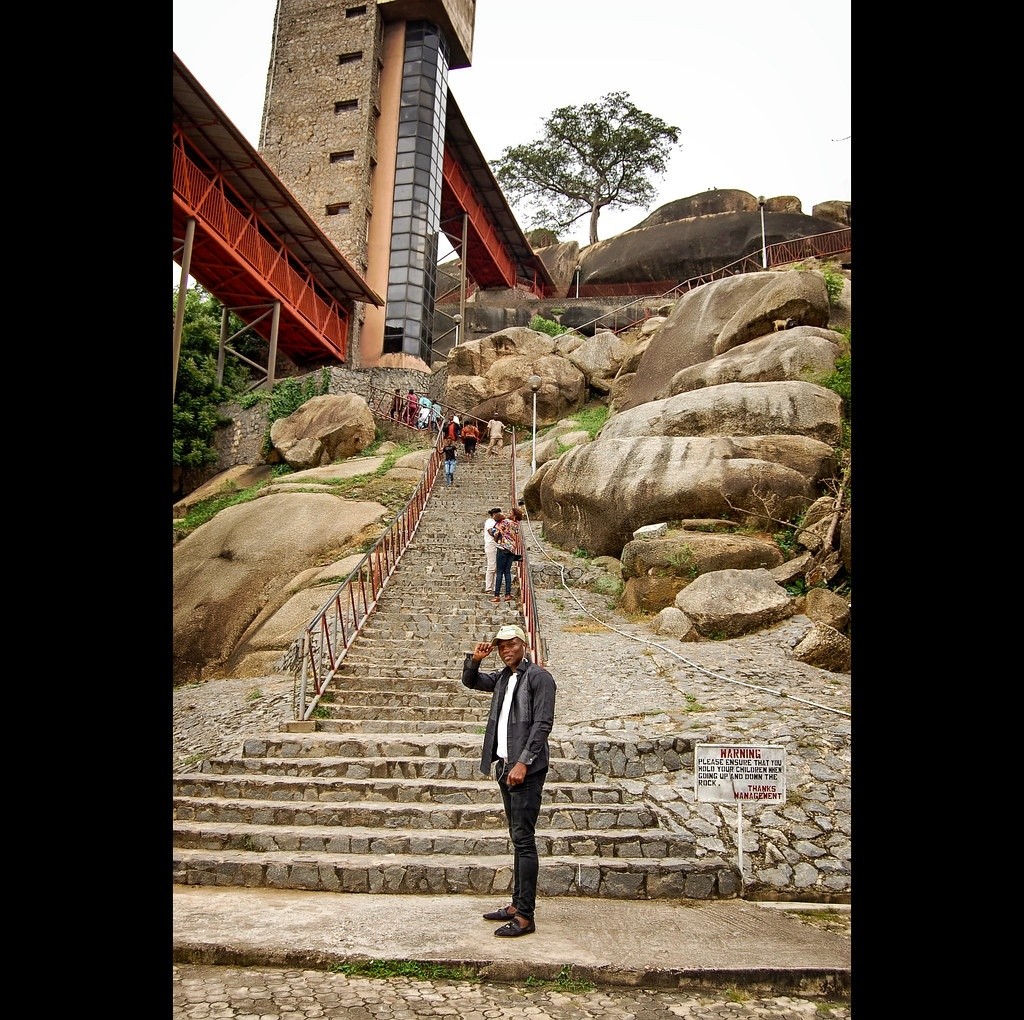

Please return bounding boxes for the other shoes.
[496,545,504,550]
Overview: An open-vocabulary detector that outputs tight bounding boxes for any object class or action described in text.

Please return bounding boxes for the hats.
[409,389,414,392]
[431,399,437,402]
[492,625,526,647]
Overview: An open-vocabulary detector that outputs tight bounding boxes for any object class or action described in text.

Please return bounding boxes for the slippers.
[486,592,492,594]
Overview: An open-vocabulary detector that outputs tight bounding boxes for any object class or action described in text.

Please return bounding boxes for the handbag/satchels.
[513,554,523,563]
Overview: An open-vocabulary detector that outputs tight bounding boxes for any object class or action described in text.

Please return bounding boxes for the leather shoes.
[482,906,521,922]
[493,919,536,938]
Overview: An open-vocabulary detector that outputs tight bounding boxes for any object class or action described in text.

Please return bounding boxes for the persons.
[487,412,511,457]
[461,421,479,464]
[431,437,457,485]
[390,389,403,422]
[484,508,501,595]
[442,411,462,446]
[462,625,556,936]
[493,507,521,602]
[403,389,442,430]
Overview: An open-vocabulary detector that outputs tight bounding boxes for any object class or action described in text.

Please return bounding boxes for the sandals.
[504,595,511,601]
[490,598,501,602]
